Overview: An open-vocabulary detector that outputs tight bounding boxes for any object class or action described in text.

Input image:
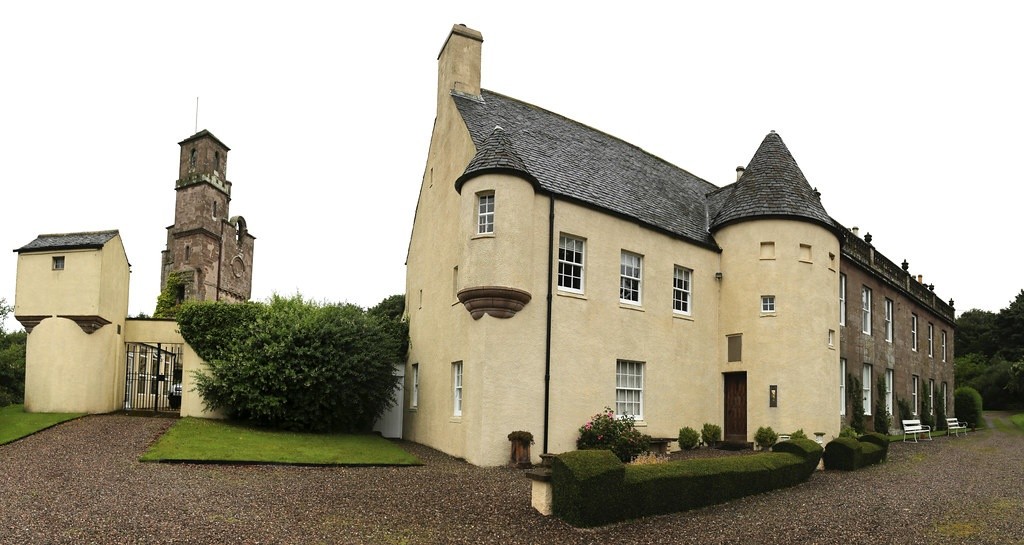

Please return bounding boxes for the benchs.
[648,438,675,454]
[945,417,969,438]
[902,419,932,444]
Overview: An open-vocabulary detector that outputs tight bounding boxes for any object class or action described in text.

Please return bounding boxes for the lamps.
[716,272,722,278]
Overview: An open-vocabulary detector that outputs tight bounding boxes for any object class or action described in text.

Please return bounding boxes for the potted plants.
[508,431,536,464]
[814,431,826,443]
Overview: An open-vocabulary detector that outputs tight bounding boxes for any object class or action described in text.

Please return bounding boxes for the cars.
[168,379,183,409]
